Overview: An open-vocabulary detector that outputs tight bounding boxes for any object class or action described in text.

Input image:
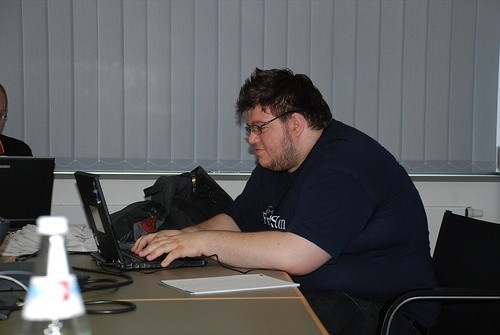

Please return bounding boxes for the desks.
[0,252,329,335]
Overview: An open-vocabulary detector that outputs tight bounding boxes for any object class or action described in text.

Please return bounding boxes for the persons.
[0,83,33,157]
[130,64,434,334]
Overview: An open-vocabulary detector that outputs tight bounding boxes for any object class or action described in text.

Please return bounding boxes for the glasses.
[244,110,305,136]
[0,113,8,121]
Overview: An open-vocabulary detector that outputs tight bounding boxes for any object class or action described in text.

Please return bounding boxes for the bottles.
[17,216,89,335]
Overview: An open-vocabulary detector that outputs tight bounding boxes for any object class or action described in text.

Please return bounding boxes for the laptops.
[0,156,55,229]
[74,171,208,271]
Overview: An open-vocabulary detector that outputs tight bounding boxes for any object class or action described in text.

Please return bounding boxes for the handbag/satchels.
[110,200,168,239]
[144,165,234,231]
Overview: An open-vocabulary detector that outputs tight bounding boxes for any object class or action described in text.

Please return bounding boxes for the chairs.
[378,210,500,335]
